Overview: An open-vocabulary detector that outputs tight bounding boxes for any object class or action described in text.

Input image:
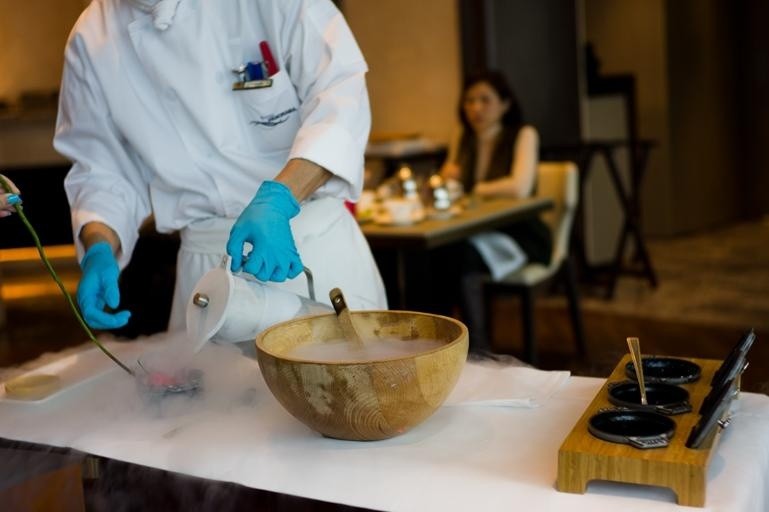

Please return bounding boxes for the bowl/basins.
[133,366,204,416]
[253,310,469,443]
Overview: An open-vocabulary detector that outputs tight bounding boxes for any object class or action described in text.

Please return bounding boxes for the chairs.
[450,161,584,360]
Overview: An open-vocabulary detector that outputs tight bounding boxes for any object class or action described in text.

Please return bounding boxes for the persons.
[1,175,23,217]
[52,1,390,350]
[398,72,552,355]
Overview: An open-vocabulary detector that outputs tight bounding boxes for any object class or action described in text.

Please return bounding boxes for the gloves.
[225,180,304,283]
[75,241,131,330]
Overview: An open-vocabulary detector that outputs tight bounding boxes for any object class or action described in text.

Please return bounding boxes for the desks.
[352,195,550,357]
